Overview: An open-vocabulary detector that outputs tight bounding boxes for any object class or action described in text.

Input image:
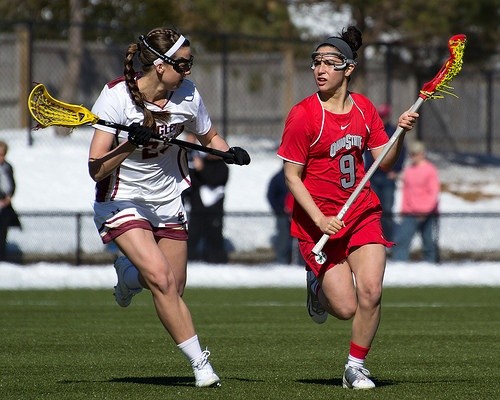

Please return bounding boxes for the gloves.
[128,122,152,148]
[223,146,251,165]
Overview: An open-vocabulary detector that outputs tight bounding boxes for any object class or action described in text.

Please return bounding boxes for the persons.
[354,104,404,256]
[275,25,419,392]
[186,149,228,264]
[394,140,441,265]
[0,142,22,262]
[267,153,290,264]
[88,27,251,388]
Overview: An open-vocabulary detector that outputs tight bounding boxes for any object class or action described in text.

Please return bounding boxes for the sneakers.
[341,364,377,390]
[190,356,221,388]
[113,255,143,306]
[305,267,329,325]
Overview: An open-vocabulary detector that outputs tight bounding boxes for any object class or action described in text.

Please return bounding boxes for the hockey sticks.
[311,34,467,256]
[28,81,235,159]
